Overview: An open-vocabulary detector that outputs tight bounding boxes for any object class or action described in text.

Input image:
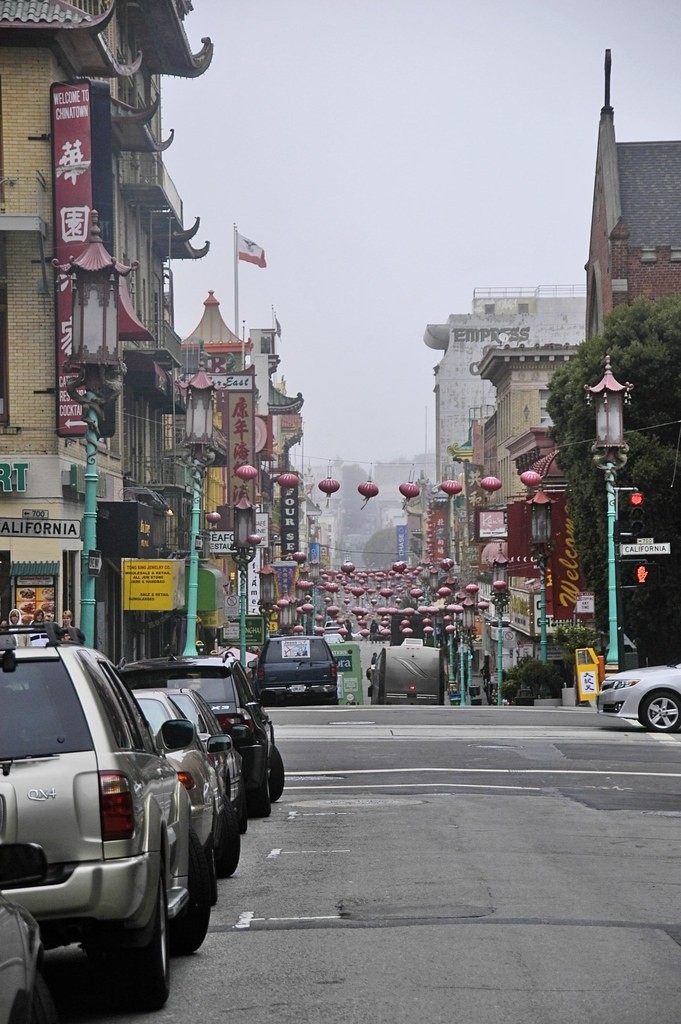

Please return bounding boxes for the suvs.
[0,617,444,1023]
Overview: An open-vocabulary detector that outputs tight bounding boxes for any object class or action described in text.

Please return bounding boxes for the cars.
[595,663,681,733]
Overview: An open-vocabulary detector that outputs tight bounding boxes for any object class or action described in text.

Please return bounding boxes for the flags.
[237,232,267,268]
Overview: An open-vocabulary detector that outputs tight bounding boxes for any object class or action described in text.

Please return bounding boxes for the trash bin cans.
[575,648,599,700]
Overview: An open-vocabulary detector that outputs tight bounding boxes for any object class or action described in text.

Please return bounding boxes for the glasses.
[37,613,43,616]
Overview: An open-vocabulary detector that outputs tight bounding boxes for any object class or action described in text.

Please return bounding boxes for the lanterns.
[205,464,541,637]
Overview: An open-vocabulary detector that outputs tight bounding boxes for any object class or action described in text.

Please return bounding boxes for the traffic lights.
[630,492,646,538]
[633,563,659,586]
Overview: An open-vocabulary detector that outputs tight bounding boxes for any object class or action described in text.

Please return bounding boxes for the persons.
[0,609,86,645]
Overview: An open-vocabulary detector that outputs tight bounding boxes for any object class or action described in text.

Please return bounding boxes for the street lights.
[526,483,560,667]
[492,541,510,706]
[179,358,223,657]
[232,478,259,670]
[433,593,479,706]
[580,352,635,674]
[47,206,138,652]
